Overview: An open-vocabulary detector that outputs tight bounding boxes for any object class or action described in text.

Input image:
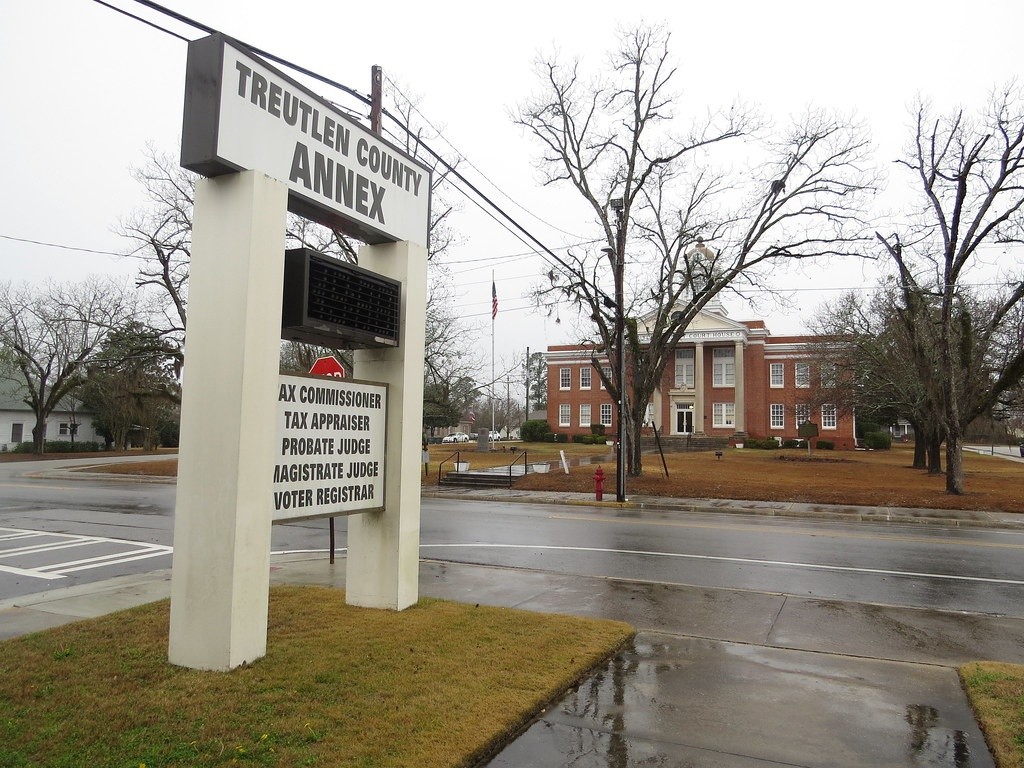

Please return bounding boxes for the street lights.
[599,245,627,504]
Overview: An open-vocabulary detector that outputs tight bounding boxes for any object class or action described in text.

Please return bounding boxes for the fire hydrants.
[593,465,606,502]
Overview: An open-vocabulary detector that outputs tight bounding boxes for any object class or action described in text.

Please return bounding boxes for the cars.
[488,431,500,442]
[442,432,470,443]
